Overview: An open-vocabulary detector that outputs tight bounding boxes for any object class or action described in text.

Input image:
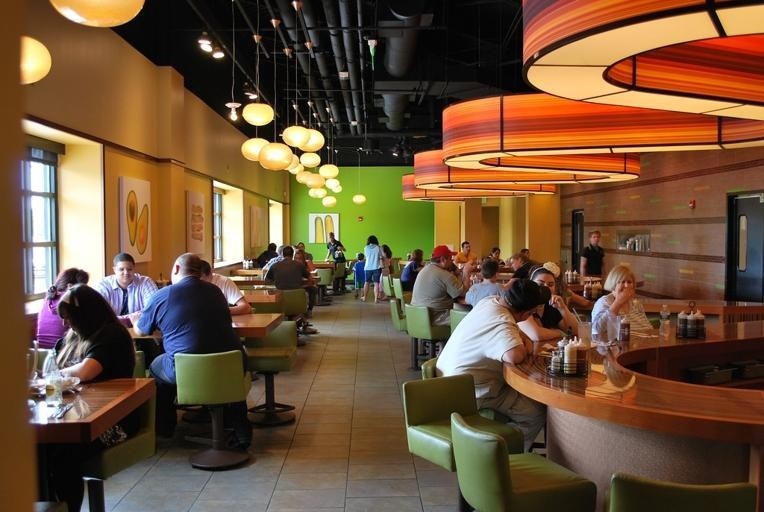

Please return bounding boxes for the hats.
[356,252,364,259]
[431,245,458,259]
[505,277,552,310]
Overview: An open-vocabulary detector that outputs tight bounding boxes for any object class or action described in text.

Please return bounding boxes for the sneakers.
[361,296,366,302]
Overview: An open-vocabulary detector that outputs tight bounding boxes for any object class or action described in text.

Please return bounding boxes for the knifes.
[56,401,73,419]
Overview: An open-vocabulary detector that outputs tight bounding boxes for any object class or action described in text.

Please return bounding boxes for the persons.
[91,252,159,320]
[127,251,253,440]
[196,260,252,318]
[253,242,318,335]
[589,262,653,344]
[29,284,134,512]
[352,235,393,303]
[432,279,552,454]
[412,241,589,326]
[35,266,134,349]
[512,268,579,342]
[400,249,424,292]
[324,232,347,294]
[578,231,603,280]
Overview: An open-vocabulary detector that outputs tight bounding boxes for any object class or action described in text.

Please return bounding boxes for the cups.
[577,322,592,348]
[607,316,620,342]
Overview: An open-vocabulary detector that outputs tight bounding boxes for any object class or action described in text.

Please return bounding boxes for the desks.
[28,376,156,445]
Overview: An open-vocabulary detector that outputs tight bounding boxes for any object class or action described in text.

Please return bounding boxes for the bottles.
[564,270,579,284]
[469,274,481,286]
[583,281,602,299]
[676,308,704,338]
[620,315,630,341]
[550,335,585,375]
[242,259,253,270]
[625,236,644,252]
[41,349,64,406]
[659,305,669,321]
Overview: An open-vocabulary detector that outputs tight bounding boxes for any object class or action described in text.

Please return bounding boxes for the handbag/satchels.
[378,246,386,268]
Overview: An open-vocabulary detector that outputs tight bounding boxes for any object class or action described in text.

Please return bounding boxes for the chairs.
[609,470,758,511]
[29,347,155,512]
[173,349,252,470]
[381,260,645,373]
[402,373,524,471]
[127,261,335,426]
[450,412,597,510]
[421,357,510,424]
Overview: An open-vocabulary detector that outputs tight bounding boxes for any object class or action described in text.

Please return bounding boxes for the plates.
[41,376,80,395]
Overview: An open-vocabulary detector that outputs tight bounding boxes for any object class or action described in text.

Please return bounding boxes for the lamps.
[198,1,366,208]
[49,1,145,28]
[20,36,52,87]
